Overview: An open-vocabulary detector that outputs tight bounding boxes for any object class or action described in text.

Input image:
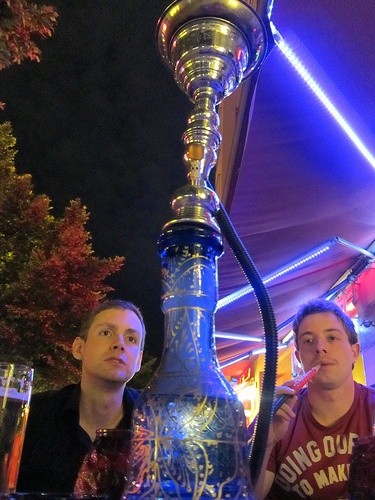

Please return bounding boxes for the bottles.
[122,224,257,500]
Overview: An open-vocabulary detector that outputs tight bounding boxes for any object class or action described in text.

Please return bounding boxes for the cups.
[0,362,34,500]
[74,429,132,500]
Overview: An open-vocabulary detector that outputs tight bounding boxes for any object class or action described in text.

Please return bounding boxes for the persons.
[248,300,375,500]
[13,300,145,500]
